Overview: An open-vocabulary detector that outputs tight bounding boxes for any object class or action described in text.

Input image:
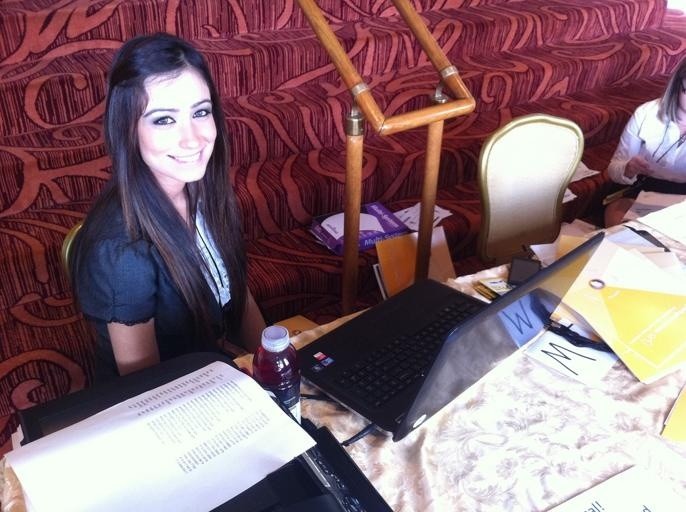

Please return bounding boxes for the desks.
[0,198,685,512]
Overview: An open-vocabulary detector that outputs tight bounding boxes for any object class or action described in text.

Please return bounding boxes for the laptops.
[296,230,606,444]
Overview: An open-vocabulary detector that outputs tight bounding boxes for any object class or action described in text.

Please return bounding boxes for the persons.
[606,55,686,198]
[71,30,267,380]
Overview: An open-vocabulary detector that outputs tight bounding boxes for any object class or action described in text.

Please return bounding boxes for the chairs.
[61,219,82,283]
[477,113,585,268]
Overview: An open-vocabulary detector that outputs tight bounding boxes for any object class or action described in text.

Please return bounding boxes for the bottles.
[249,325,302,427]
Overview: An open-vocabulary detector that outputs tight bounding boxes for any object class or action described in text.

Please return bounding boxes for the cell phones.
[505,256,541,284]
